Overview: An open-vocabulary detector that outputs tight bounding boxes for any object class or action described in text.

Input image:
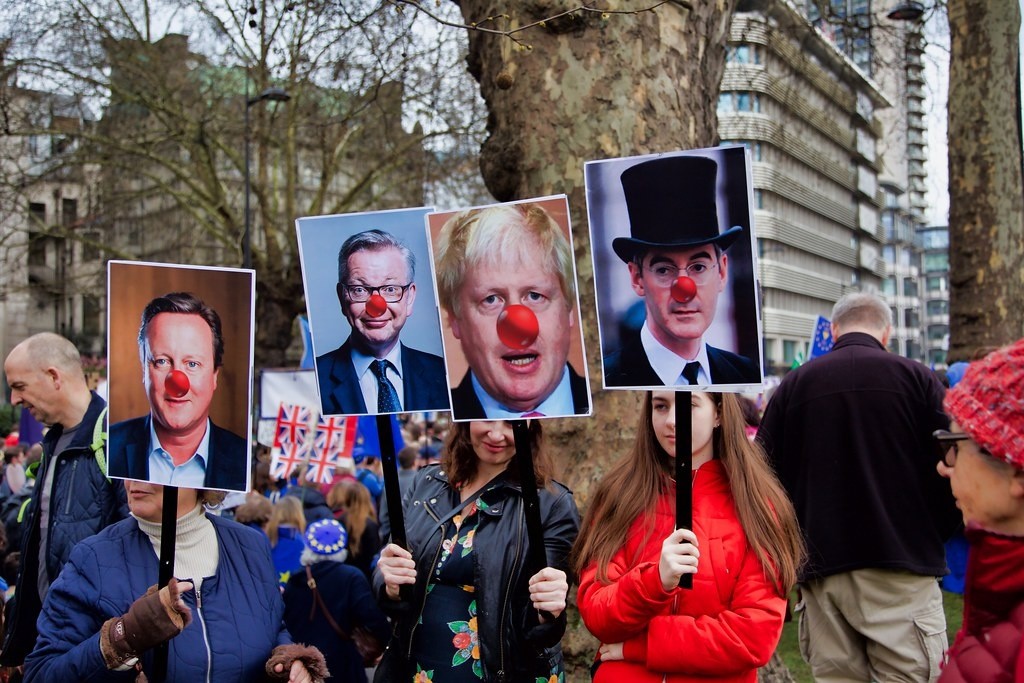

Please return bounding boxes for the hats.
[612,155,743,263]
[352,447,364,464]
[942,338,1024,469]
[300,518,346,565]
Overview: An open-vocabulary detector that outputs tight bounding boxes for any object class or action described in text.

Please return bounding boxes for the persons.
[569,388,807,682]
[736,360,974,593]
[316,228,451,416]
[371,420,580,683]
[22,479,332,683]
[109,293,247,493]
[936,337,1024,683]
[0,331,451,683]
[433,202,589,421]
[754,291,948,683]
[604,156,762,387]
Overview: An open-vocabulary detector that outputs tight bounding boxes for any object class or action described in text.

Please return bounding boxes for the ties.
[371,359,403,413]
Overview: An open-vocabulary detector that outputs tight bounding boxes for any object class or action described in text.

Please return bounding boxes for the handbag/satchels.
[353,632,383,660]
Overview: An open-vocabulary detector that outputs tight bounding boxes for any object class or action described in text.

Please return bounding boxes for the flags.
[352,413,406,470]
[295,315,317,370]
[810,315,835,360]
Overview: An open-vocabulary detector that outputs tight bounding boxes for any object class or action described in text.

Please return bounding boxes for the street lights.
[245,90,291,270]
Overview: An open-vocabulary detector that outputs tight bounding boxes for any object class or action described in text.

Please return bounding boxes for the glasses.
[934,429,972,468]
[639,260,719,288]
[340,281,411,303]
